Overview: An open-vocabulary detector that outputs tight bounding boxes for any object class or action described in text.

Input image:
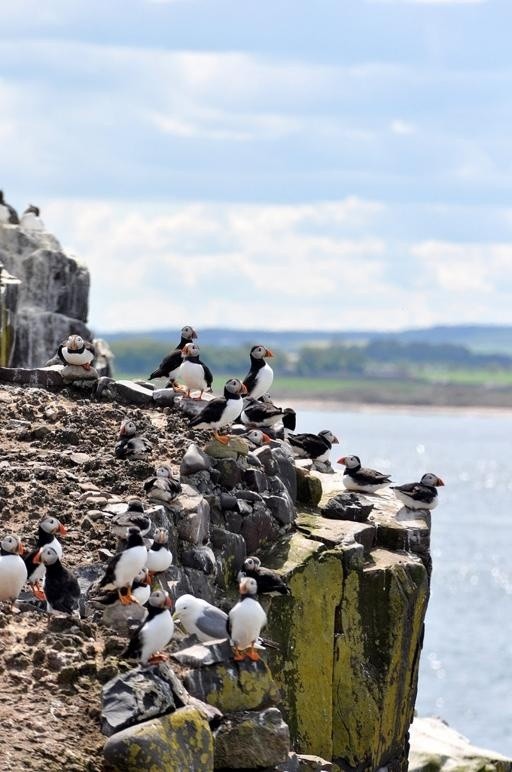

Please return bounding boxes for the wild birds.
[94,497,173,606]
[389,472,444,511]
[236,554,294,598]
[115,588,176,666]
[57,334,96,372]
[115,419,153,462]
[142,463,183,504]
[147,325,295,447]
[225,576,267,663]
[0,513,83,624]
[172,593,282,653]
[0,190,21,226]
[286,428,339,459]
[18,204,43,232]
[336,455,393,493]
